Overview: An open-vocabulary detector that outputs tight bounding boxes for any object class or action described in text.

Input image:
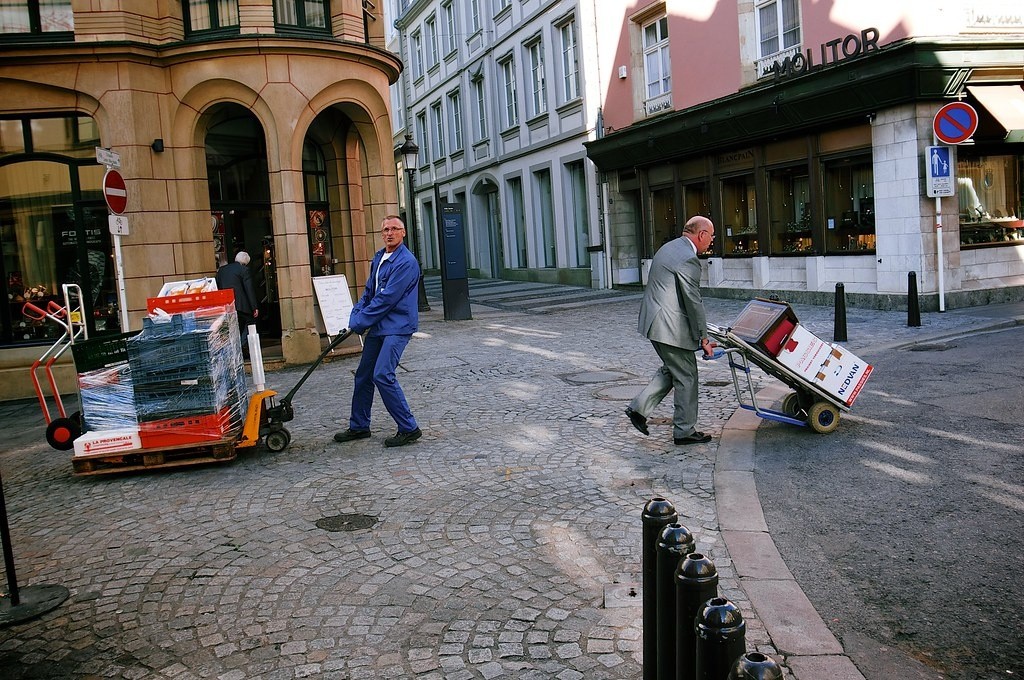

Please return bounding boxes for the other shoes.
[334,428,371,443]
[385,427,422,447]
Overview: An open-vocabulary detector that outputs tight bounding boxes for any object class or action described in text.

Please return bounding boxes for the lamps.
[152,139,164,152]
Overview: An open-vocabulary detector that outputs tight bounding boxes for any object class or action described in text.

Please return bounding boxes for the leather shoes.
[674,431,712,445]
[625,407,649,436]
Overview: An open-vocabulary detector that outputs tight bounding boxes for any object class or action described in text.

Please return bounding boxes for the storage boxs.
[811,341,874,408]
[728,296,800,363]
[776,322,833,382]
[70,277,251,458]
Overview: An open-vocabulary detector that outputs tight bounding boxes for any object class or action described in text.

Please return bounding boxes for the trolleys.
[702,322,852,434]
[21,283,354,477]
[21,284,88,452]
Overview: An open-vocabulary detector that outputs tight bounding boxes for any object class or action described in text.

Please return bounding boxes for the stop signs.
[102,169,128,217]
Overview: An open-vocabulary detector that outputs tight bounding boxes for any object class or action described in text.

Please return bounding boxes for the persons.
[625,215,714,445]
[333,215,423,448]
[214,251,259,359]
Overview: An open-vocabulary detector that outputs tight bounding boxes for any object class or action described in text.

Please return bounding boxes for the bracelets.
[704,339,710,346]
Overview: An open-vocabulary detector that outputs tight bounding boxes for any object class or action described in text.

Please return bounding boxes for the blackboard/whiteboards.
[311,274,354,337]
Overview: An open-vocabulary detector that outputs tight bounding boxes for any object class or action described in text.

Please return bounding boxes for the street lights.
[399,134,431,313]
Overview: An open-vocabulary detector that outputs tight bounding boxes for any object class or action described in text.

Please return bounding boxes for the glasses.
[702,229,716,242]
[380,227,404,232]
